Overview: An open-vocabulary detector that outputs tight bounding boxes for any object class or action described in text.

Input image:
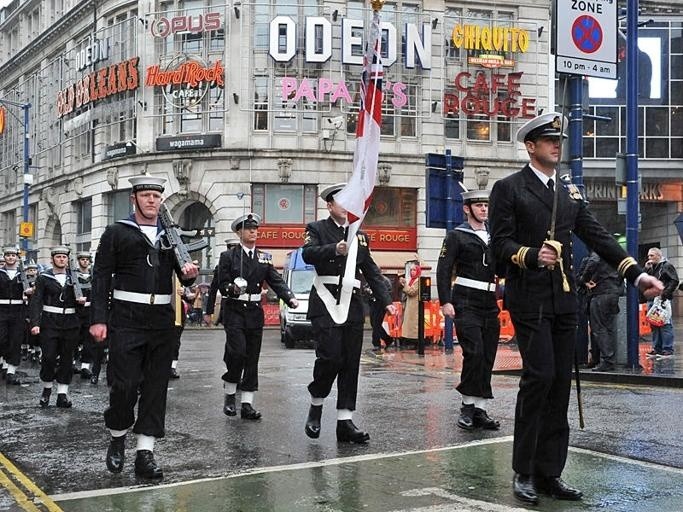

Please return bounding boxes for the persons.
[488,112,665,506]
[639,245,681,360]
[216,214,299,418]
[1,238,623,385]
[88,175,199,479]
[301,184,398,444]
[25,246,80,408]
[435,189,507,430]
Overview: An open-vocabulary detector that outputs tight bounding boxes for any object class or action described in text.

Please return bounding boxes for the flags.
[334,7,383,251]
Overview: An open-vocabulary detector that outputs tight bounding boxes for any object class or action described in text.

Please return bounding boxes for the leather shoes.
[241,403,261,419]
[106,433,124,473]
[513,472,539,506]
[537,477,583,499]
[583,361,608,371]
[73,364,97,384]
[56,393,72,407]
[21,347,42,365]
[40,387,51,405]
[224,393,236,416]
[170,368,180,379]
[0,364,20,385]
[135,450,163,479]
[337,420,370,442]
[306,405,322,437]
[373,339,394,350]
[458,402,500,430]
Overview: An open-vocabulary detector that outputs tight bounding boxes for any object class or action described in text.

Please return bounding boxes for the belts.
[0,296,28,304]
[113,289,171,304]
[84,302,90,307]
[42,305,76,315]
[455,276,497,292]
[318,276,361,289]
[223,294,262,302]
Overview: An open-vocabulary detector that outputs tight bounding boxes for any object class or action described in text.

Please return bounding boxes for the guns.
[18,258,37,292]
[158,203,209,273]
[67,245,92,300]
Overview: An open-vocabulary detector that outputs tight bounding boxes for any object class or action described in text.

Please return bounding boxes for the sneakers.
[646,349,673,357]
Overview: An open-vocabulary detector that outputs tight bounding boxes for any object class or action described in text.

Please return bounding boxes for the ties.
[339,226,344,236]
[547,178,556,195]
[249,250,253,259]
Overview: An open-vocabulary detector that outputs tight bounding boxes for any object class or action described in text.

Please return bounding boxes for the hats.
[321,184,347,202]
[460,190,492,205]
[231,213,262,232]
[225,239,242,247]
[129,178,167,192]
[77,253,92,259]
[517,113,568,144]
[0,247,38,271]
[49,247,73,257]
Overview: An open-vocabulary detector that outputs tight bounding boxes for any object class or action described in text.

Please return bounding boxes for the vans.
[277,251,324,349]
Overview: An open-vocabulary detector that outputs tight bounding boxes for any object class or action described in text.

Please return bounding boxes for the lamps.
[106,168,118,187]
[475,167,490,190]
[277,159,292,180]
[377,163,392,183]
[72,178,82,195]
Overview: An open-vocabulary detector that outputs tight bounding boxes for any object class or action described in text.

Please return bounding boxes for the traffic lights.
[419,277,430,301]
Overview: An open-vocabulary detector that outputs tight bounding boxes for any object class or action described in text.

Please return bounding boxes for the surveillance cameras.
[328,116,345,123]
